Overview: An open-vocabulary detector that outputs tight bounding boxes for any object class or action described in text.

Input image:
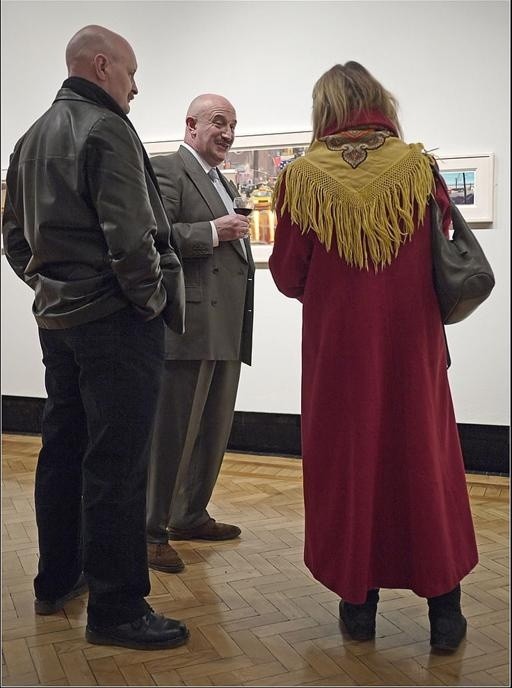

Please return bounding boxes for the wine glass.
[232,196,255,239]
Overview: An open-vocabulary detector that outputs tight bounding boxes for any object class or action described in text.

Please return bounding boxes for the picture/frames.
[141,131,321,263]
[435,154,495,228]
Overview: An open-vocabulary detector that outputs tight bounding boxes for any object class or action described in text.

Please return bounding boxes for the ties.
[208,167,237,215]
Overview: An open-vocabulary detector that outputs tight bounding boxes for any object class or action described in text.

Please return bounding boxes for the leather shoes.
[86,602,189,650]
[147,542,184,573]
[169,517,241,541]
[34,572,89,616]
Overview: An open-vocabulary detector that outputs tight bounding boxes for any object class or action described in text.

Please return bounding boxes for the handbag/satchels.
[430,196,494,324]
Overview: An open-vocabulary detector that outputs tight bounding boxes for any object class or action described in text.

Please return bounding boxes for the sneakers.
[339,597,376,640]
[429,614,467,651]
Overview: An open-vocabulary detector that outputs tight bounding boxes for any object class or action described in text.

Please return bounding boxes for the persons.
[2,25,190,650]
[268,61,480,651]
[144,93,257,573]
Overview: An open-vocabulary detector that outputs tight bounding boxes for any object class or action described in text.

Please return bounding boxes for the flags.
[272,154,295,170]
